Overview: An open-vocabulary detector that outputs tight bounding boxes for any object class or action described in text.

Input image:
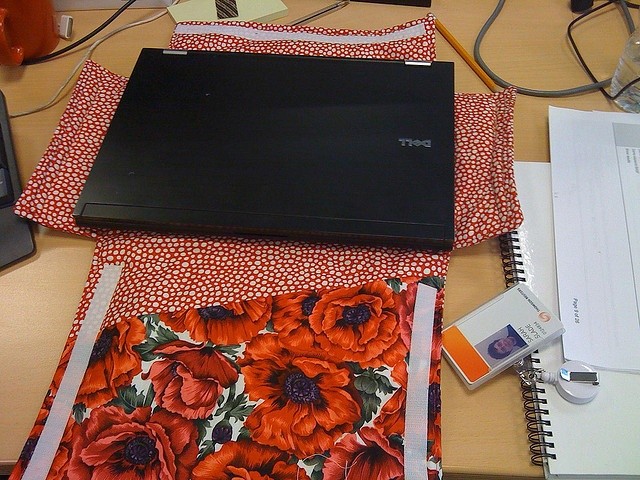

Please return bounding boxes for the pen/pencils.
[427,12,503,92]
[288,1,351,26]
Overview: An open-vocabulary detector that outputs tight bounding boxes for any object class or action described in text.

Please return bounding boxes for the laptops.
[73,47,455,249]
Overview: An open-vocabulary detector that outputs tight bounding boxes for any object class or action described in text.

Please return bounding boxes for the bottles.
[610,25,640,114]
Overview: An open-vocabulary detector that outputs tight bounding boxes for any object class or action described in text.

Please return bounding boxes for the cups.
[0,0,58,68]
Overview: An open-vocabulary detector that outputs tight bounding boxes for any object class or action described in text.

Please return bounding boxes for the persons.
[488,325,526,359]
